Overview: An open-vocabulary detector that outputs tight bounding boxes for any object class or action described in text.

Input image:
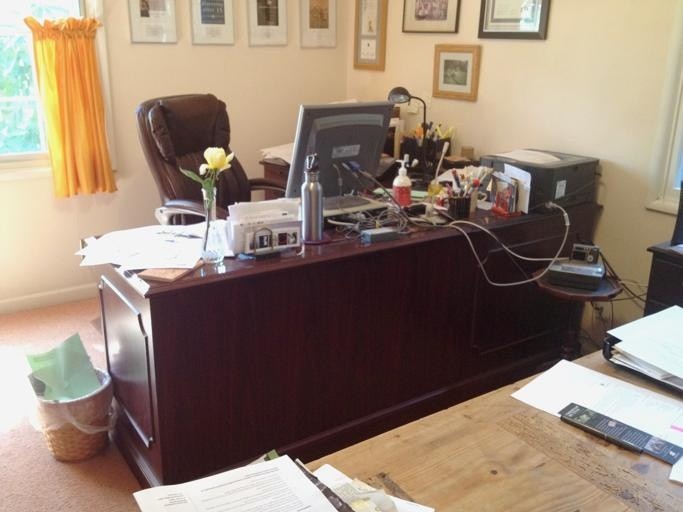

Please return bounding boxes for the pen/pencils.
[445,169,481,199]
[427,120,455,139]
[174,231,203,239]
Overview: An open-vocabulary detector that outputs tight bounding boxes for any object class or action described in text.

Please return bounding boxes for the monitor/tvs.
[284,100,395,198]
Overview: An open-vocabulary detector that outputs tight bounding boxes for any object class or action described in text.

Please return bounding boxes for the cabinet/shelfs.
[258,138,395,203]
[642,236,683,321]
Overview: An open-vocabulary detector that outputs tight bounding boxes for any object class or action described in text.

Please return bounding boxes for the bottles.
[298,152,324,243]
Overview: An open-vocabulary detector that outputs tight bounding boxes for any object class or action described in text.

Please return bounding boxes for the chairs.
[134,91,291,229]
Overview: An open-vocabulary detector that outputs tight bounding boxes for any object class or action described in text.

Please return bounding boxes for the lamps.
[386,85,431,169]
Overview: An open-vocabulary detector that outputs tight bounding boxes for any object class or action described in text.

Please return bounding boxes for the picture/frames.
[477,2,551,40]
[189,1,235,47]
[431,43,482,102]
[401,1,461,34]
[353,1,388,73]
[127,2,177,44]
[246,2,289,48]
[298,2,336,48]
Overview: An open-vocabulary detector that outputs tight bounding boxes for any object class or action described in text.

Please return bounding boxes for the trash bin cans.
[23,366,112,462]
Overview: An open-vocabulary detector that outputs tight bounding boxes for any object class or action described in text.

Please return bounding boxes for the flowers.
[178,144,236,210]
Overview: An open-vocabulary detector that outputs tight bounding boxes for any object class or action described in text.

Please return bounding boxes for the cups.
[441,196,469,220]
[459,192,487,213]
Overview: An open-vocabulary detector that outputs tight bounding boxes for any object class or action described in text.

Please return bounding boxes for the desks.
[78,202,605,496]
[295,336,683,512]
[528,267,626,368]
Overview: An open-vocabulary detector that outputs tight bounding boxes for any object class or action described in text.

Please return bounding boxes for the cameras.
[570,243,599,265]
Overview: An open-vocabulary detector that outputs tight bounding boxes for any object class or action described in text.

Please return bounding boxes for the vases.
[193,187,229,264]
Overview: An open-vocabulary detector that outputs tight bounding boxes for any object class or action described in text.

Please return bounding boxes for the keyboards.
[298,195,368,209]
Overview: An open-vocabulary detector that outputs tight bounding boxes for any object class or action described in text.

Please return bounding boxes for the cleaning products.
[390,158,412,207]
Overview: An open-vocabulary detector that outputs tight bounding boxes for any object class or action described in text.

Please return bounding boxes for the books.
[132,448,431,512]
[610,344,673,380]
[137,257,203,285]
